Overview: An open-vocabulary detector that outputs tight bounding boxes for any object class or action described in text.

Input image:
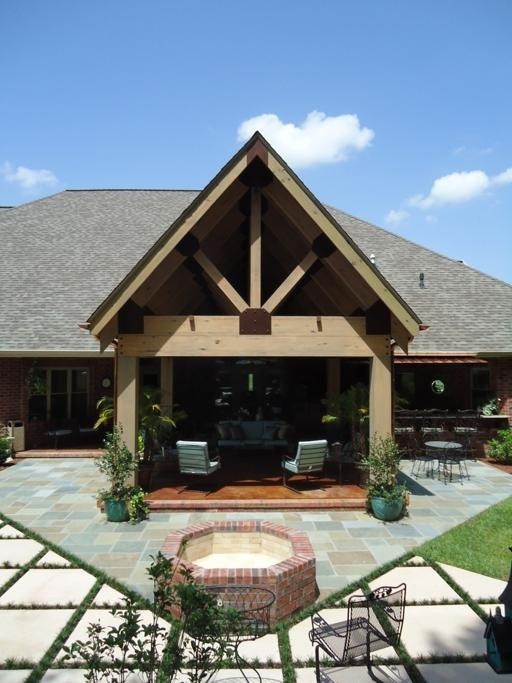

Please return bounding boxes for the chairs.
[175,439,220,496]
[280,439,332,494]
[413,431,478,485]
[309,583,407,683]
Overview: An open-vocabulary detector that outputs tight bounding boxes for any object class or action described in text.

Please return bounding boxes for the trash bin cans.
[8,420,26,450]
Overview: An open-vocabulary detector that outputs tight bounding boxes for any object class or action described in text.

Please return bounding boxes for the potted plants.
[322,385,367,484]
[358,430,410,521]
[93,389,176,490]
[96,421,152,525]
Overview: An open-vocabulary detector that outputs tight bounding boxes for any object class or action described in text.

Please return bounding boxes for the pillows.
[215,421,289,440]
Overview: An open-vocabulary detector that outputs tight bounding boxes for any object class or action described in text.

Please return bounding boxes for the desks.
[188,585,276,677]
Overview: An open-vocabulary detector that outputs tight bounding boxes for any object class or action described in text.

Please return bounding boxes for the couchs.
[216,420,290,449]
